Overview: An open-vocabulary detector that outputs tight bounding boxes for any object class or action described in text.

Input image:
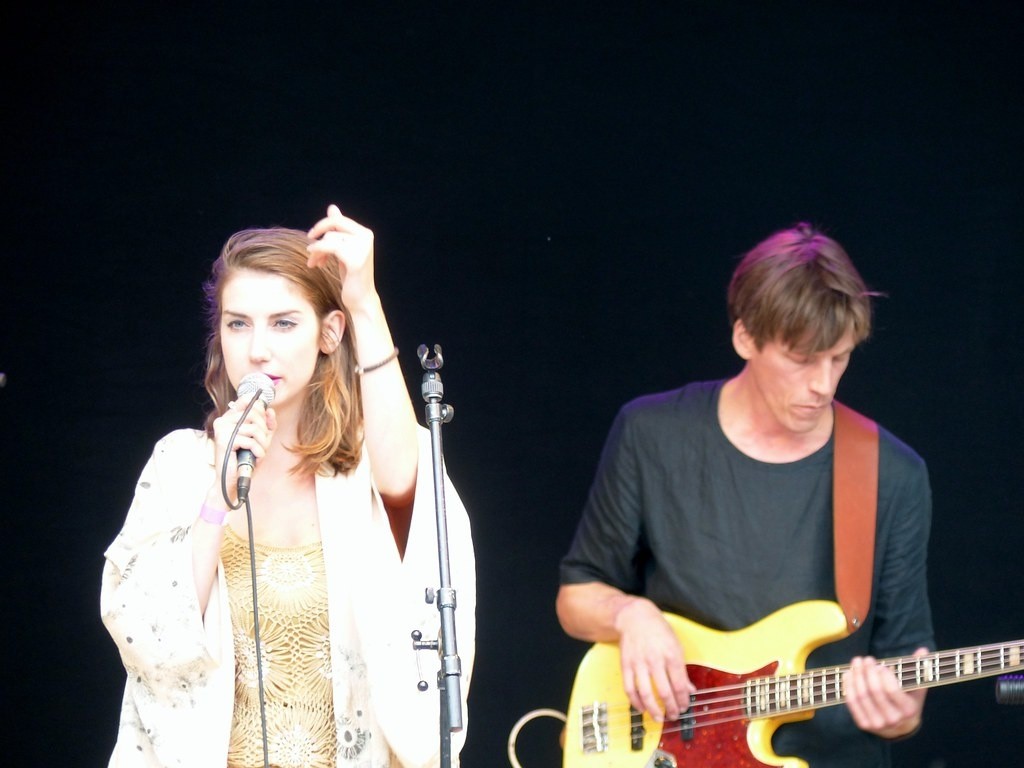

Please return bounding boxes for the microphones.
[236,371,276,501]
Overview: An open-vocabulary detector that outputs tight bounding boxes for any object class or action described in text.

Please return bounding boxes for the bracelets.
[351,347,398,377]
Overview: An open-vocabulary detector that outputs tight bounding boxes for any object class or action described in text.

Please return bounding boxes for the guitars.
[564,599,1023,767]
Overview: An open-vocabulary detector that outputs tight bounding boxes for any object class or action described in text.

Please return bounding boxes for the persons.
[553,224,943,768]
[95,202,473,768]
